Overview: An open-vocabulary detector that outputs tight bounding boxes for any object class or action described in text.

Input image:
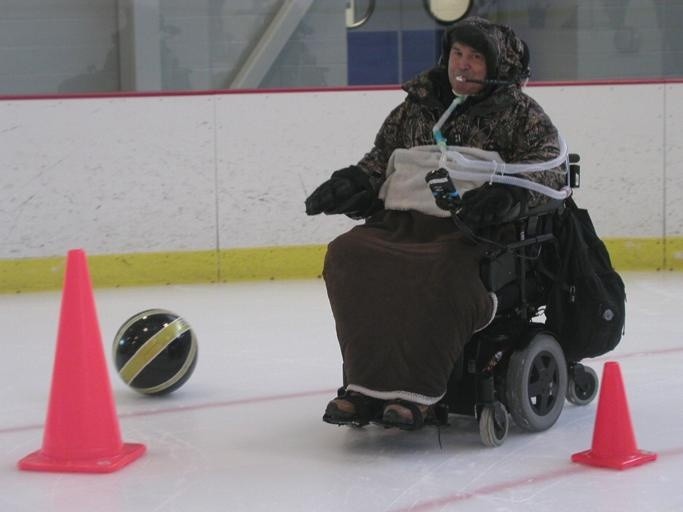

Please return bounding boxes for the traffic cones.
[18,249,147,473]
[570,362,656,471]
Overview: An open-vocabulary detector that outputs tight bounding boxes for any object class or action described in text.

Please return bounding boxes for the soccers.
[112,309,198,397]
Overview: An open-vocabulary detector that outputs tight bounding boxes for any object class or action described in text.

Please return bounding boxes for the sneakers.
[382,397,429,424]
[324,392,377,420]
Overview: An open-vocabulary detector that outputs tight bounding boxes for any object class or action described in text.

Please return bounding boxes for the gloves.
[459,183,514,223]
[304,173,360,216]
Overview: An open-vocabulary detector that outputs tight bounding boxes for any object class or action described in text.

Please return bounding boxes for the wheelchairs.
[323,153,599,448]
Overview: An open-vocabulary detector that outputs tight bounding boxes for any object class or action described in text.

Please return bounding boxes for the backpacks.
[542,192,627,363]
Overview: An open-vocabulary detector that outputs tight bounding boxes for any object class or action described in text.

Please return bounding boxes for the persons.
[302,16,571,432]
